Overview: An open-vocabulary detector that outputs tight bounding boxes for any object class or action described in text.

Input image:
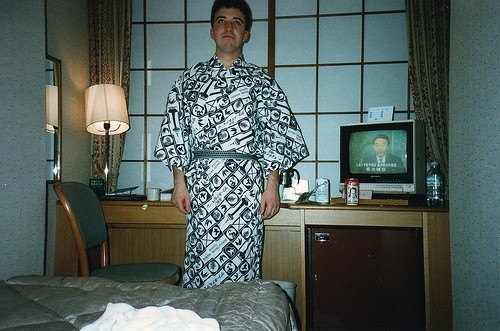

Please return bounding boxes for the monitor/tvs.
[338,118,428,200]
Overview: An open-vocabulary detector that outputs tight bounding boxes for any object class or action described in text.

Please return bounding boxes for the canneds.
[315,178,329,204]
[344,178,359,205]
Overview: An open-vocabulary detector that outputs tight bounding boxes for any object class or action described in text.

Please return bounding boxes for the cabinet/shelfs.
[55,199,453,331]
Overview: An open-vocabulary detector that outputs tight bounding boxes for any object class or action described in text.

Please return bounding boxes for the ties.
[379,157,384,170]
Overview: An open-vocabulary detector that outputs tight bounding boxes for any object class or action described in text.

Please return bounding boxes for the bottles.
[425,162,445,209]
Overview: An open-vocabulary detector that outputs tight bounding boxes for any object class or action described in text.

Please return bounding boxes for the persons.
[154,0,310,290]
[358,134,406,173]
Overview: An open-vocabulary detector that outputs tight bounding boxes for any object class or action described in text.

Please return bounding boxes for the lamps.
[84,84,147,202]
[45,84,60,179]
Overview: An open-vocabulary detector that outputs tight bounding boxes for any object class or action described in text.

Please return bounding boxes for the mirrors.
[45,52,62,184]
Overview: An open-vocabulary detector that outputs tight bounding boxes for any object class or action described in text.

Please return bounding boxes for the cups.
[147,187,160,201]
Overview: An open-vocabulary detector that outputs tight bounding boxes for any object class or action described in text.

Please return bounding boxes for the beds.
[0,275,300,331]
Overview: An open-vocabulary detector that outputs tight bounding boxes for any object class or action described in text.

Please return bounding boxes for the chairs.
[53,181,183,286]
